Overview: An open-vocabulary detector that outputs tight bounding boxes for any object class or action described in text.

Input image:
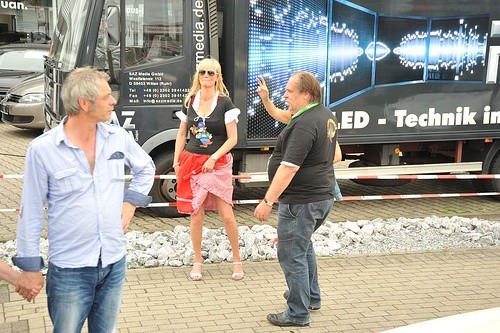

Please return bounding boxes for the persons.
[253,72,343,326]
[0,66,156,333]
[171,59,244,281]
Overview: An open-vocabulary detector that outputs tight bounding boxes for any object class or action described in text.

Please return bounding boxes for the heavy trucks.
[44,0,500,218]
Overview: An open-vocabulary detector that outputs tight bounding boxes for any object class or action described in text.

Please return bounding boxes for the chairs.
[134,34,173,64]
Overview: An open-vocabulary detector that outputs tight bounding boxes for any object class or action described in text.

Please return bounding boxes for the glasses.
[198,70,219,76]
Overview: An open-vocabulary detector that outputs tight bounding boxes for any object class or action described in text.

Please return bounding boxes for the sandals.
[189,263,203,281]
[230,262,244,280]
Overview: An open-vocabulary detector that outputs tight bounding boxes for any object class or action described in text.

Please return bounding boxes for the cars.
[0,28,52,102]
[0,70,44,130]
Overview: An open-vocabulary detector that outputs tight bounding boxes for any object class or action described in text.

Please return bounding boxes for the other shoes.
[266,312,310,327]
[284,290,321,310]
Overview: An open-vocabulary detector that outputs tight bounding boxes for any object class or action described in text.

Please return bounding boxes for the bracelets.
[172,163,180,168]
[211,154,218,161]
[263,198,274,207]
